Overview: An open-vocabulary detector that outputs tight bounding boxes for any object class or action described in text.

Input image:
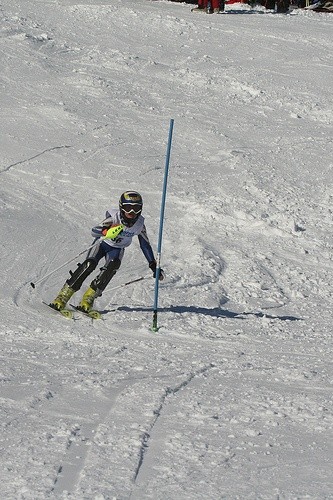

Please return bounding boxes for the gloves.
[150,262,164,281]
[101,224,123,243]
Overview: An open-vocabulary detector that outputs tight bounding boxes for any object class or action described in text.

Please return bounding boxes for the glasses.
[120,203,142,215]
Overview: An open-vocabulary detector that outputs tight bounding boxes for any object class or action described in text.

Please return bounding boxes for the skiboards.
[69,303,105,320]
[42,301,75,320]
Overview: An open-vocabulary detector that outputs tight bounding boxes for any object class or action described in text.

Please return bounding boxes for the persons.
[50,190,165,312]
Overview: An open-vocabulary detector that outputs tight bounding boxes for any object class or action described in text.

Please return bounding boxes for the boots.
[50,281,70,309]
[79,287,97,313]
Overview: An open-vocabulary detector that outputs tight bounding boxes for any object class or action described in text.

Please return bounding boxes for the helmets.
[119,190,143,227]
